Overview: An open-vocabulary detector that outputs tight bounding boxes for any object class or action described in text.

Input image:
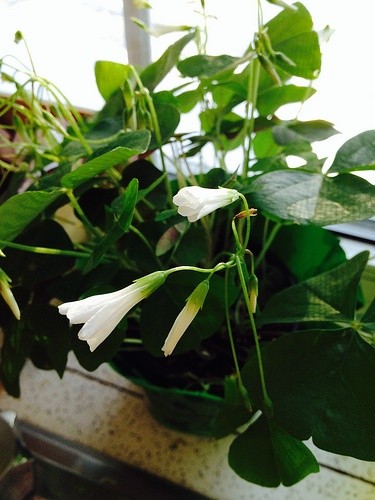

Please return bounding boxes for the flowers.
[3,0,372,486]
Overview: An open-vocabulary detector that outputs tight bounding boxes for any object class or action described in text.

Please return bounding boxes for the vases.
[104,345,259,441]
